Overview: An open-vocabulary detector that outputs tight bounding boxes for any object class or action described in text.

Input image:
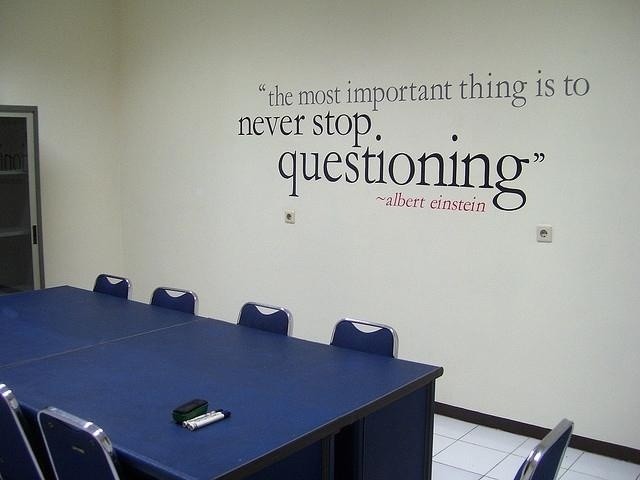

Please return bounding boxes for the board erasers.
[173,399,209,422]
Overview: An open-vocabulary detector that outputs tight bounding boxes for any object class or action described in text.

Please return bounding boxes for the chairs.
[237,301,294,338]
[514,417,575,480]
[93,274,133,301]
[332,318,397,358]
[0,380,125,480]
[149,286,199,316]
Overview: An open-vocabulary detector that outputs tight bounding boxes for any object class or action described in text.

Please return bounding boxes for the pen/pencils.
[183,407,230,434]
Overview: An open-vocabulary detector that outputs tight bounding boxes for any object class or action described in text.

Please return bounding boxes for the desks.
[0,285,444,480]
